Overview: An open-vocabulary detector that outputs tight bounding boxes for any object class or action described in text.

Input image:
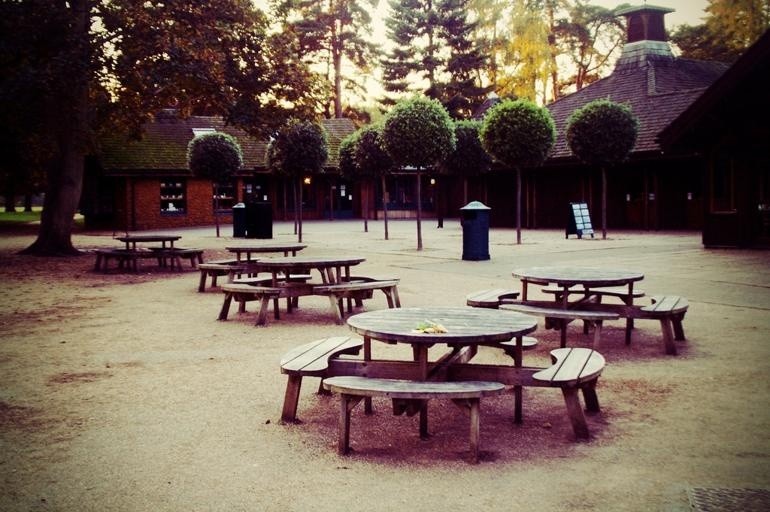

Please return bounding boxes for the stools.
[315,279,400,322]
[161,248,206,272]
[640,295,690,356]
[91,248,155,274]
[532,348,606,440]
[209,256,257,284]
[541,286,644,329]
[448,336,537,424]
[217,284,282,327]
[233,272,312,314]
[323,374,504,465]
[466,289,520,309]
[197,264,246,293]
[500,303,620,352]
[281,337,364,421]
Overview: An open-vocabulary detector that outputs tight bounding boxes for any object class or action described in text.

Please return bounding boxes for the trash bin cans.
[460,201,492,260]
[232,202,247,238]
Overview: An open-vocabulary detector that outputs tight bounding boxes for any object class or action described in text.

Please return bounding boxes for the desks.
[227,244,307,282]
[348,306,538,437]
[116,235,181,271]
[256,257,366,319]
[512,267,644,349]
